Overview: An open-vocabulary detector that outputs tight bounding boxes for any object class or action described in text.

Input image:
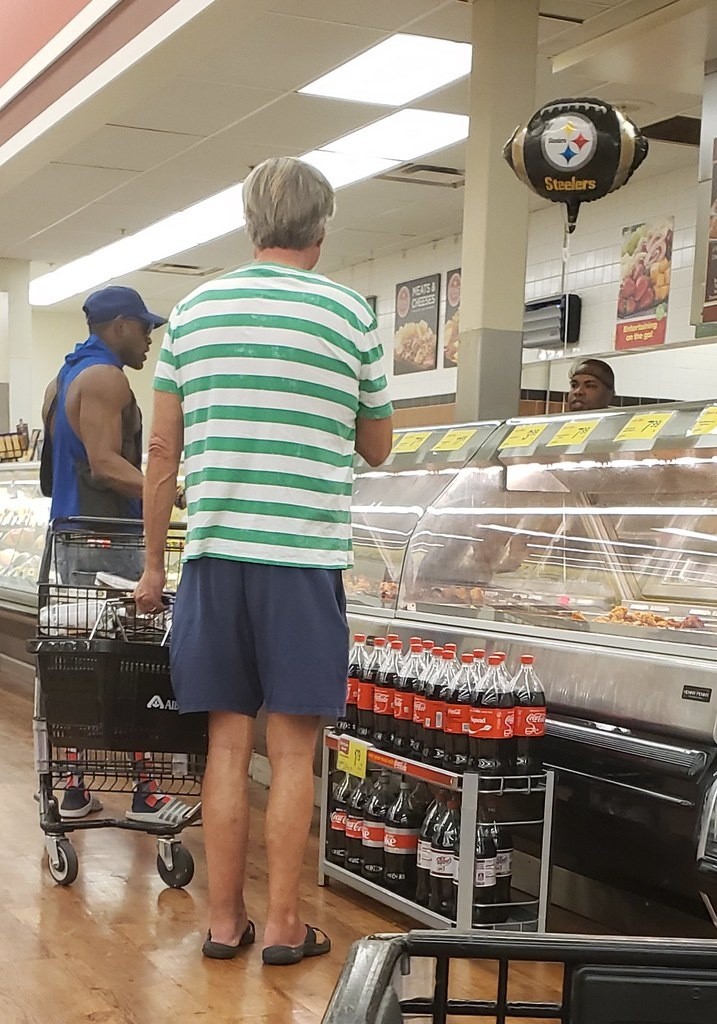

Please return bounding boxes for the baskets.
[25,595,210,755]
[321,928,717,1024]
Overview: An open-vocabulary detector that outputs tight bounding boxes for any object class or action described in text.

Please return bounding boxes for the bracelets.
[175,486,185,511]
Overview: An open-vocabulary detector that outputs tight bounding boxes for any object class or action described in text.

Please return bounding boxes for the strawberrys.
[618,261,656,315]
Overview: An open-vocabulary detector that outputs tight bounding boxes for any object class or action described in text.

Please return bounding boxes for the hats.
[82,285,168,329]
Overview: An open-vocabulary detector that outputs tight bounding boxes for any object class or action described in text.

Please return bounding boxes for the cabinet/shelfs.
[318,726,554,929]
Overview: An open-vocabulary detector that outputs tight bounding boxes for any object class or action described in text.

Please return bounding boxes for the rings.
[148,607,157,612]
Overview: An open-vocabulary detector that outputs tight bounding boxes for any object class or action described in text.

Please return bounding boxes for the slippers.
[202,919,255,958]
[262,923,331,965]
[58,792,103,817]
[125,797,202,825]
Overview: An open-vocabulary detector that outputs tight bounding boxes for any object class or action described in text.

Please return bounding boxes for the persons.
[569,358,615,412]
[41,285,203,827]
[134,158,393,965]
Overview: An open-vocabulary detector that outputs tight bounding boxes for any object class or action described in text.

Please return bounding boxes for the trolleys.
[25,514,210,890]
[319,929,717,1024]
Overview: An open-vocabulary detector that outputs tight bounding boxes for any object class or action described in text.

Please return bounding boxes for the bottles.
[325,634,546,929]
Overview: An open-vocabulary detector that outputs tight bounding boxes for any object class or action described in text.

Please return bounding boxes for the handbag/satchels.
[40,432,52,497]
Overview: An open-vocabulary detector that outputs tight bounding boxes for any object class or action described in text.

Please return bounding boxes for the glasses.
[119,314,155,335]
